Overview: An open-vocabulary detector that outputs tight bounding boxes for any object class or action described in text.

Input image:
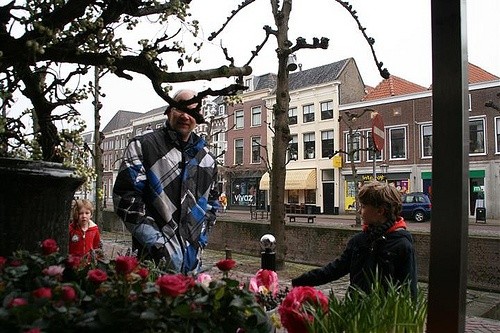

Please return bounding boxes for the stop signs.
[372,113,385,151]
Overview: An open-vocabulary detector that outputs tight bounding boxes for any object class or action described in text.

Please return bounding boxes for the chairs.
[285,203,305,214]
[250,205,256,220]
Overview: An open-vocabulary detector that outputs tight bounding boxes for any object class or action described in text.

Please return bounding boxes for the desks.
[256,210,268,219]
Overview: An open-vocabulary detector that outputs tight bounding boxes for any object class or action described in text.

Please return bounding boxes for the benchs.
[287,215,316,223]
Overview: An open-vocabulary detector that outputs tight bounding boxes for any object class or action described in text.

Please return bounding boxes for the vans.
[399,191,431,223]
